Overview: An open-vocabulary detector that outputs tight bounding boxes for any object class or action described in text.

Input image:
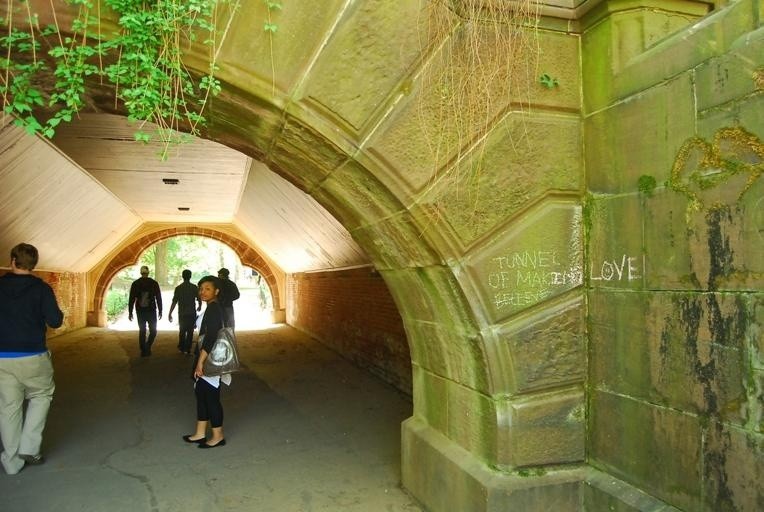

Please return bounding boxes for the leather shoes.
[199,438,225,448]
[183,435,206,443]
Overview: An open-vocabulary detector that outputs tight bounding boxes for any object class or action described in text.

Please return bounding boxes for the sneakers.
[20,454,44,464]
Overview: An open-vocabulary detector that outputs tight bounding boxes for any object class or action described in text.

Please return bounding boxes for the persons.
[183,276,229,448]
[0,243,64,475]
[128,265,162,356]
[217,268,239,328]
[168,270,202,354]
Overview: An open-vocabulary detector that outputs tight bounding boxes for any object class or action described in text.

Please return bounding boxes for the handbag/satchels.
[198,327,240,376]
